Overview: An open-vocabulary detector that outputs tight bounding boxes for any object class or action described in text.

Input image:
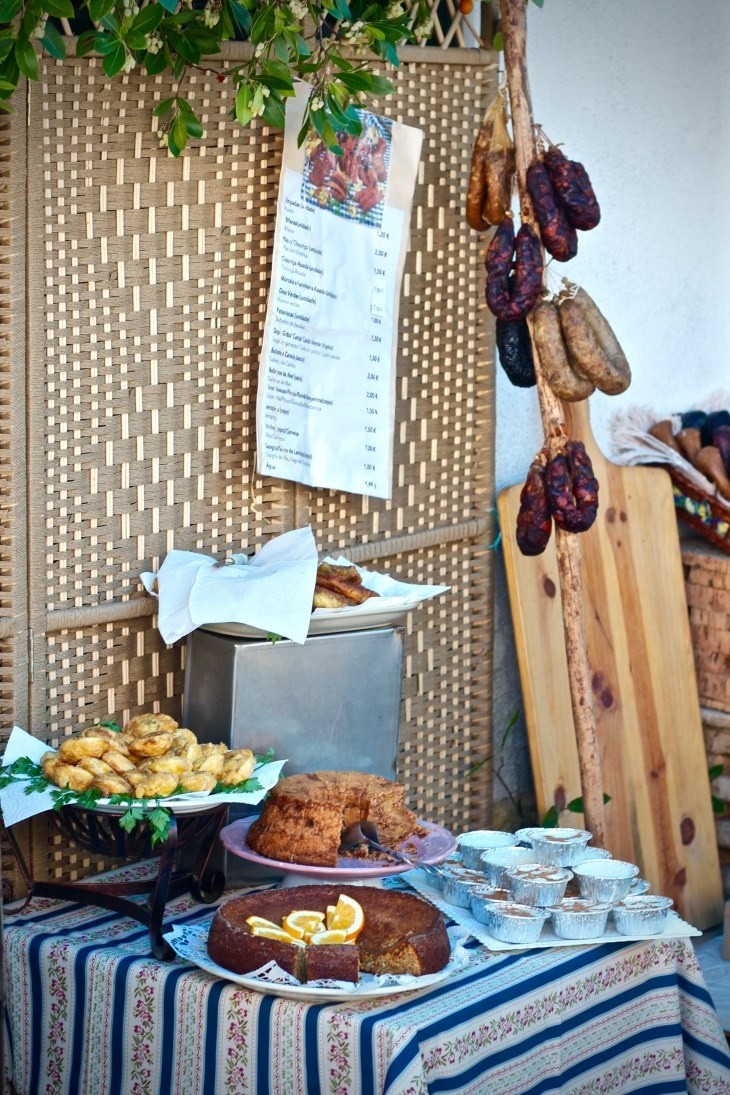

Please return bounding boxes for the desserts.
[422,827,676,944]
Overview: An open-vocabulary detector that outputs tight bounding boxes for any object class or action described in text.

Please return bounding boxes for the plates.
[161,919,465,1002]
[220,815,457,882]
[198,602,420,639]
[398,852,703,952]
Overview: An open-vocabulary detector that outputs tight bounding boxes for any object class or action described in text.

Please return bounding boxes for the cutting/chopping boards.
[493,395,725,937]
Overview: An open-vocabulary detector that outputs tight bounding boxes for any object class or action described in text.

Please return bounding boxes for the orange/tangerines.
[247,892,365,949]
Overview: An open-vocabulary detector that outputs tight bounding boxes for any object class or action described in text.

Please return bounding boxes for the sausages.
[514,438,599,557]
[466,94,633,403]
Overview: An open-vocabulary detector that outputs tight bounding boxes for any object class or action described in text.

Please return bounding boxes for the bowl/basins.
[423,826,673,944]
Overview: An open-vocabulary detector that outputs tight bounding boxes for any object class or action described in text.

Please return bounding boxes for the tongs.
[340,819,448,882]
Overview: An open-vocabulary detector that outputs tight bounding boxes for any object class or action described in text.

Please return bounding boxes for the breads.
[246,770,426,867]
[207,883,452,983]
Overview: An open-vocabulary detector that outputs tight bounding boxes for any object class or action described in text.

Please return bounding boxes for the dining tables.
[1,843,729,1095]
